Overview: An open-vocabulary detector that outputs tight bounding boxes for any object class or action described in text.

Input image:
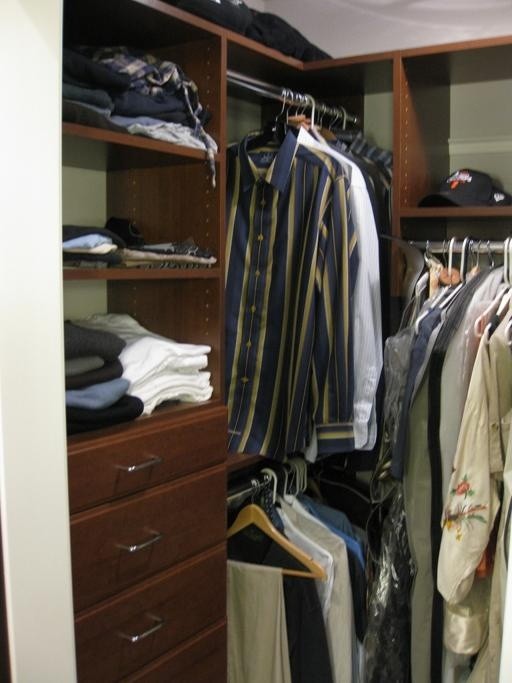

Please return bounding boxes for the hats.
[419,169,512,207]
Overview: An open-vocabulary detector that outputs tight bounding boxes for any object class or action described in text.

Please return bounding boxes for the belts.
[424,251,443,300]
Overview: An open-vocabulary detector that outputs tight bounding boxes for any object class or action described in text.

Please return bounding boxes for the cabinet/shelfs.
[62,0,223,682]
[397,34,511,683]
[224,38,397,683]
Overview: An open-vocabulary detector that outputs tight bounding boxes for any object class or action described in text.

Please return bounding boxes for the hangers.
[225,459,335,578]
[402,239,512,339]
[233,88,360,144]
